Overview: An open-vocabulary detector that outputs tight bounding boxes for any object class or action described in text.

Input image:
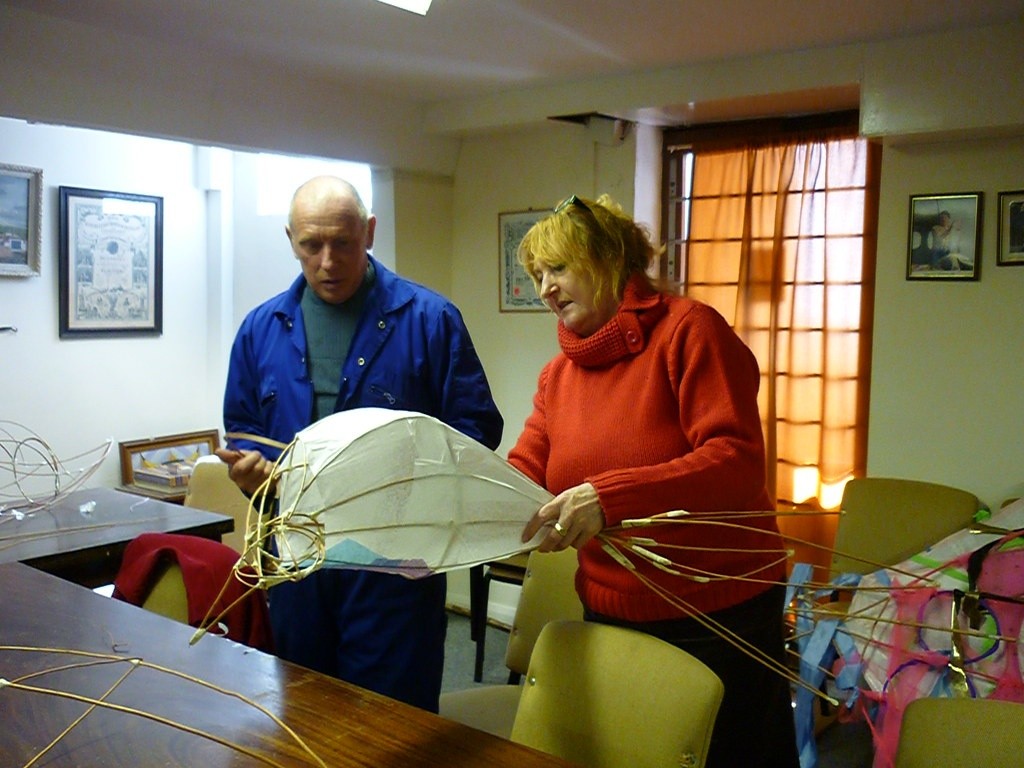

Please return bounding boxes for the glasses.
[553,194,594,216]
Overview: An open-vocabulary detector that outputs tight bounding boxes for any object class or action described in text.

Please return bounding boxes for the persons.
[213,175,506,718]
[487,193,804,768]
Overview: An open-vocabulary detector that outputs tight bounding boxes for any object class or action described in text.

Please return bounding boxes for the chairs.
[830,477,985,584]
[897,696,1024,767]
[511,622,728,768]
[439,547,584,740]
[113,534,267,651]
[180,457,274,560]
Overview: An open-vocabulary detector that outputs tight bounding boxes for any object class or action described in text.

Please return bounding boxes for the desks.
[1,561,586,768]
[2,489,236,592]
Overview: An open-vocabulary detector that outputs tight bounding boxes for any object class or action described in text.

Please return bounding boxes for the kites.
[782,494,1024,768]
[188,408,932,709]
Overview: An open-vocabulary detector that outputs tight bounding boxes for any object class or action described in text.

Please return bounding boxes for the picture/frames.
[905,192,981,281]
[997,190,1024,268]
[1,163,45,280]
[498,206,555,313]
[59,186,165,338]
[119,428,221,487]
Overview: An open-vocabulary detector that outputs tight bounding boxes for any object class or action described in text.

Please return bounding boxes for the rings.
[552,522,567,539]
